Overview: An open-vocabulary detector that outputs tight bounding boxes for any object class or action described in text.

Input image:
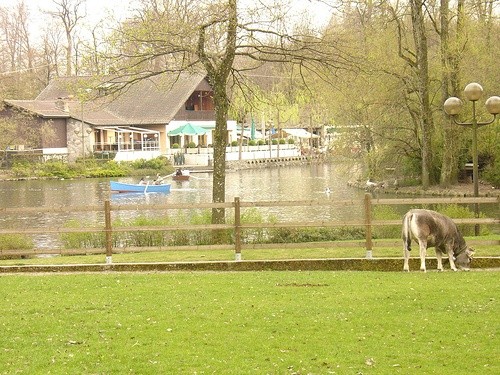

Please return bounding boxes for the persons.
[152,173,164,185]
[139,178,147,185]
[176,169,182,175]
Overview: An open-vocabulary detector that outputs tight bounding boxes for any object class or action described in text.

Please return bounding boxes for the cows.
[400,208,476,274]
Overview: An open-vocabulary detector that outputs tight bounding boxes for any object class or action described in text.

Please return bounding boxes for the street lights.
[443,82,499,235]
[265,119,277,158]
[74,126,94,166]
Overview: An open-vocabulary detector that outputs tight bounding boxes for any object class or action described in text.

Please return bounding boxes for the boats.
[172,170,190,181]
[109,181,171,193]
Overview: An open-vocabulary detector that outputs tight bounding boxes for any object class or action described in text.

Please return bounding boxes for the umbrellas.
[167,123,207,154]
